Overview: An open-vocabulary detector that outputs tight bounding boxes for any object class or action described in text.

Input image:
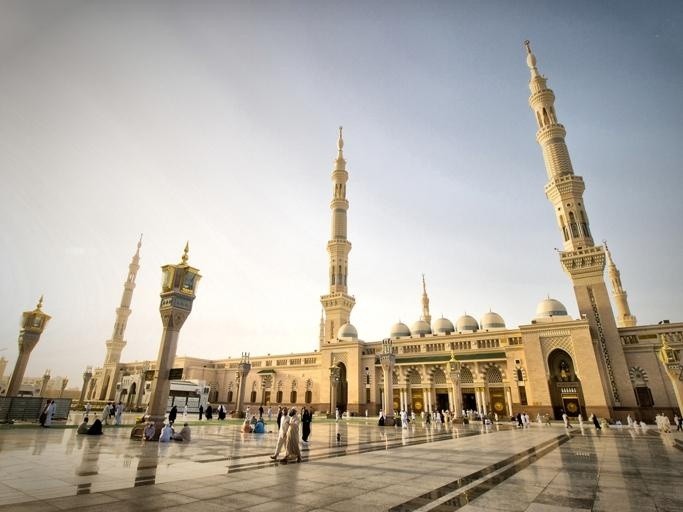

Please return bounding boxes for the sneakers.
[271,456,304,462]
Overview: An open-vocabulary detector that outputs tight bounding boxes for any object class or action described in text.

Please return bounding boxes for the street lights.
[658,331,682,415]
[446,348,466,424]
[5,291,53,395]
[125,239,203,444]
[324,357,340,420]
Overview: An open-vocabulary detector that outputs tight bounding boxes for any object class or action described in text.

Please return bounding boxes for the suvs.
[341,410,364,419]
[0,385,35,397]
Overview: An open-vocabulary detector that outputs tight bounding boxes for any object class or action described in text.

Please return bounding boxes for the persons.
[460,409,530,428]
[270,405,342,463]
[39,397,123,435]
[536,411,683,433]
[378,408,452,430]
[131,402,192,443]
[198,404,273,433]
